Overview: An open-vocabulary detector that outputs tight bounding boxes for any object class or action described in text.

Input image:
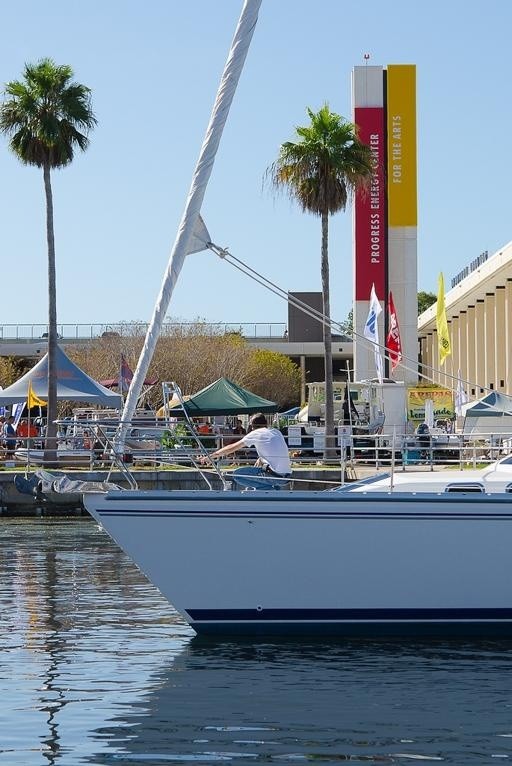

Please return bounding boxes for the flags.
[119,353,134,392]
[436,274,451,366]
[385,293,402,374]
[364,287,384,383]
[29,380,47,408]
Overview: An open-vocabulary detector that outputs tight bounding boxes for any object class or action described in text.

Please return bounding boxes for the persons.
[83,431,91,449]
[0,415,39,459]
[202,410,292,492]
[192,419,246,445]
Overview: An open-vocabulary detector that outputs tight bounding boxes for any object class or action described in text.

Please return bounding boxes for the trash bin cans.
[400,441,419,465]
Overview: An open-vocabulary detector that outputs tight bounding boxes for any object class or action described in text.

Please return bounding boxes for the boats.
[79,636,512,766]
[17,402,176,466]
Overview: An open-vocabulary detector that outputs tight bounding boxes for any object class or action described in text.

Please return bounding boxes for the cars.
[98,329,122,337]
[38,332,64,340]
[270,407,300,426]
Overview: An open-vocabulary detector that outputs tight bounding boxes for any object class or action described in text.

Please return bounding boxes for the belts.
[266,467,293,478]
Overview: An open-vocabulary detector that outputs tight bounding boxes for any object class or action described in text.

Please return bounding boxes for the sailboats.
[15,1,512,645]
